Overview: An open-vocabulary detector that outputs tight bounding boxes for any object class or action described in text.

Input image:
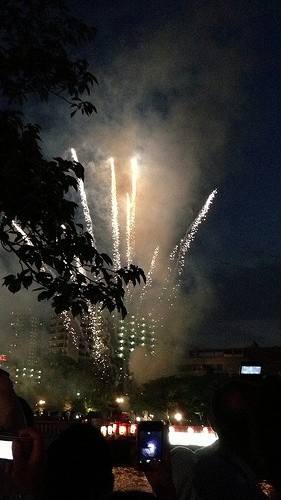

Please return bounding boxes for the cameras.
[0,432,34,463]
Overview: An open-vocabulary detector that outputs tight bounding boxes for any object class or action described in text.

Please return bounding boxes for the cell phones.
[239,362,262,377]
[134,421,164,472]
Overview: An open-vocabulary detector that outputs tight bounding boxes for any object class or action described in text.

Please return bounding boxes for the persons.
[0,368,281,500]
